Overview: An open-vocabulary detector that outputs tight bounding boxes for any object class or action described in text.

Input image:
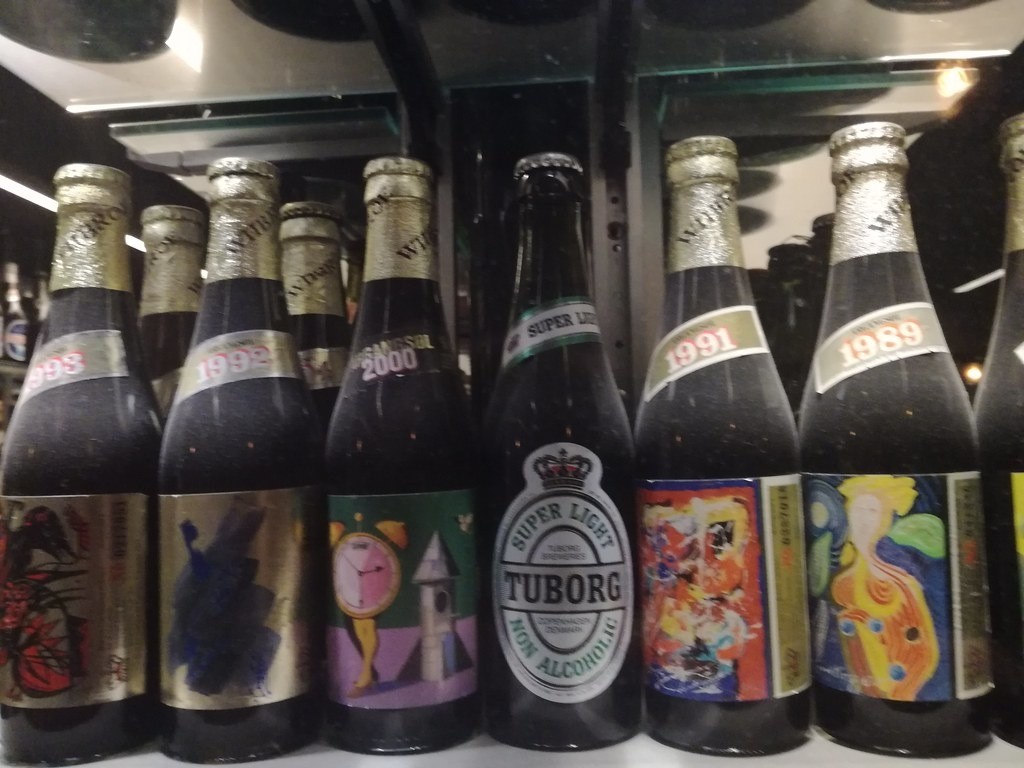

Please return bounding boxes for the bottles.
[974,114,1024,749]
[634,135,811,756]
[800,120,995,758]
[0,156,480,768]
[481,153,634,752]
